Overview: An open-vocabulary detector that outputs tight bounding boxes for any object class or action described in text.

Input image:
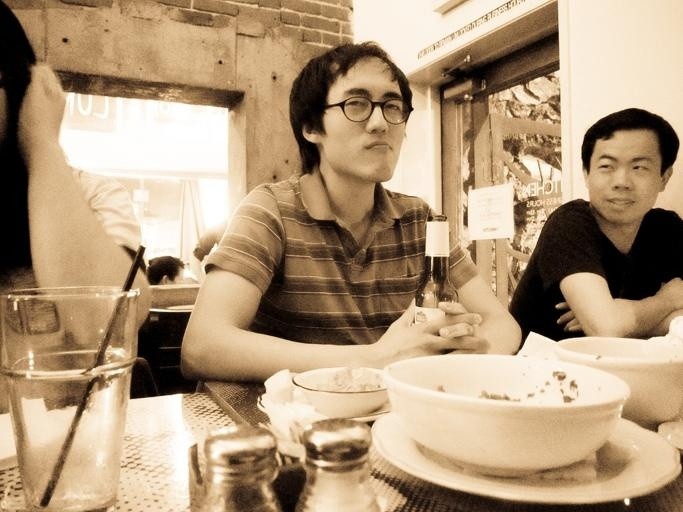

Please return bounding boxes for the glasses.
[318,96,413,124]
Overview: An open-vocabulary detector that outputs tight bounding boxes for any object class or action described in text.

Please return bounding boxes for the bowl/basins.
[553,337,683,424]
[289,365,386,413]
[380,353,630,477]
[683,427,683,429]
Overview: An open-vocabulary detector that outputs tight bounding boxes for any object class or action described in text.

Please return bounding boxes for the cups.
[0,285,140,511]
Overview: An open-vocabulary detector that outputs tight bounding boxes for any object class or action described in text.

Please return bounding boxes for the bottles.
[414,214,461,324]
[195,420,380,512]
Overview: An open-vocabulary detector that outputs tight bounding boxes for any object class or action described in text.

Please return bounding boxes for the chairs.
[130,357,159,399]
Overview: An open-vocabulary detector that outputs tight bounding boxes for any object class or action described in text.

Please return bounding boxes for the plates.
[372,422,682,506]
[258,393,392,422]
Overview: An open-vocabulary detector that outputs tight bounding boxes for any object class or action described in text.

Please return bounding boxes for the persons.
[179,40,524,381]
[508,107,682,337]
[0,1,155,419]
[146,254,184,284]
